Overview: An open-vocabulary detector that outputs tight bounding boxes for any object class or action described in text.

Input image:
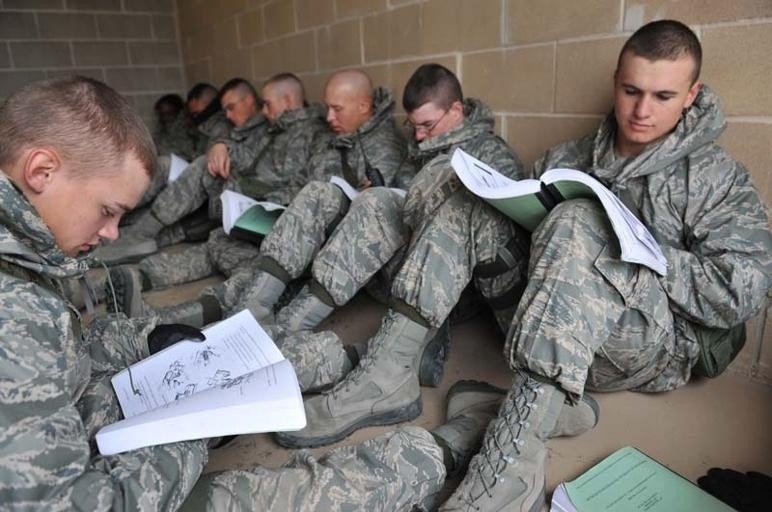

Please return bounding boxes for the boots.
[273,306,428,448]
[434,372,568,511]
[104,265,203,332]
[273,282,334,344]
[429,379,598,481]
[223,272,284,332]
[354,310,450,387]
[90,213,164,264]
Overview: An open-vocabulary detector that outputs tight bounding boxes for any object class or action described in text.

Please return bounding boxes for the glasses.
[216,95,254,114]
[403,106,451,134]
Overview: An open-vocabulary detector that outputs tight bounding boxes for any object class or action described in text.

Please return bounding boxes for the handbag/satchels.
[692,321,746,377]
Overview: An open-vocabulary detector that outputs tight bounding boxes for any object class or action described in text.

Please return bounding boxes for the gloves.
[149,323,205,354]
[697,467,771,511]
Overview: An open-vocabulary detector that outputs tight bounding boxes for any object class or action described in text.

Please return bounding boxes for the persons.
[1,20,771,512]
